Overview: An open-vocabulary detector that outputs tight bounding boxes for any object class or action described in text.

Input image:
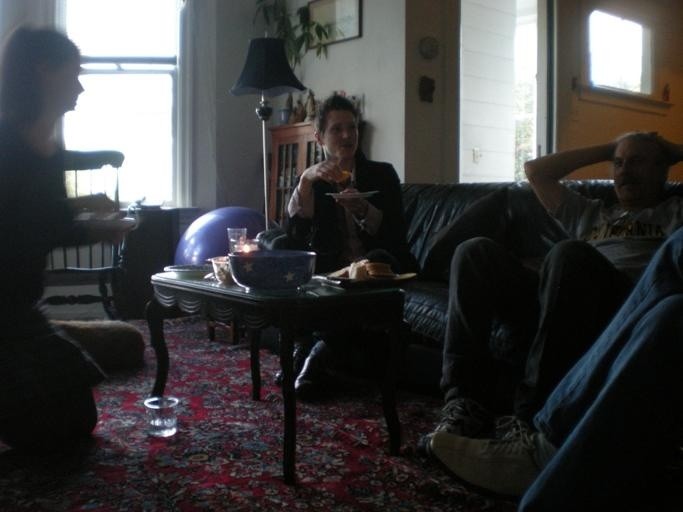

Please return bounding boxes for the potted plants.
[248,1,345,123]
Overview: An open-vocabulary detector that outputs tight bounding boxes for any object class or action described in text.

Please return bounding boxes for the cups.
[210,257,234,287]
[226,227,247,255]
[143,397,178,438]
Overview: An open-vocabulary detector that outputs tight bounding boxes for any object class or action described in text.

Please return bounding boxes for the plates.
[328,273,396,289]
[74,215,136,233]
[325,191,377,200]
[163,264,214,280]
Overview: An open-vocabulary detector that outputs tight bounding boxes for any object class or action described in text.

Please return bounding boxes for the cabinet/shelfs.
[268,121,360,228]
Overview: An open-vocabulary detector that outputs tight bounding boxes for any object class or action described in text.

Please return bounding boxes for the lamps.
[227,37,305,227]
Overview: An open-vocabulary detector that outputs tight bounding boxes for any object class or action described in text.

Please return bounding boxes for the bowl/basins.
[229,249,317,297]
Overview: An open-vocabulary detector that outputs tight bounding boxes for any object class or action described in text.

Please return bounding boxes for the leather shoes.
[293,349,324,397]
[275,342,306,385]
[426,423,543,502]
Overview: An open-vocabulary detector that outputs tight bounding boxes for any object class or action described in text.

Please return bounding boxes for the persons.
[416,123,683,459]
[0,20,138,460]
[270,94,408,389]
[430,224,683,511]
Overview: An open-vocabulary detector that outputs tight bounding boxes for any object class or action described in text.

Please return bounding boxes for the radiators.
[45,240,118,272]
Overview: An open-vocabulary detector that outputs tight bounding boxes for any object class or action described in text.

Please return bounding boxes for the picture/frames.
[305,0,361,50]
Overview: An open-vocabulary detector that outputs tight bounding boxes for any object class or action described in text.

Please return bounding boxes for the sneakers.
[417,399,483,457]
[496,415,531,439]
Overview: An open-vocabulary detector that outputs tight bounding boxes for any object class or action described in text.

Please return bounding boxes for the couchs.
[374,175,681,388]
